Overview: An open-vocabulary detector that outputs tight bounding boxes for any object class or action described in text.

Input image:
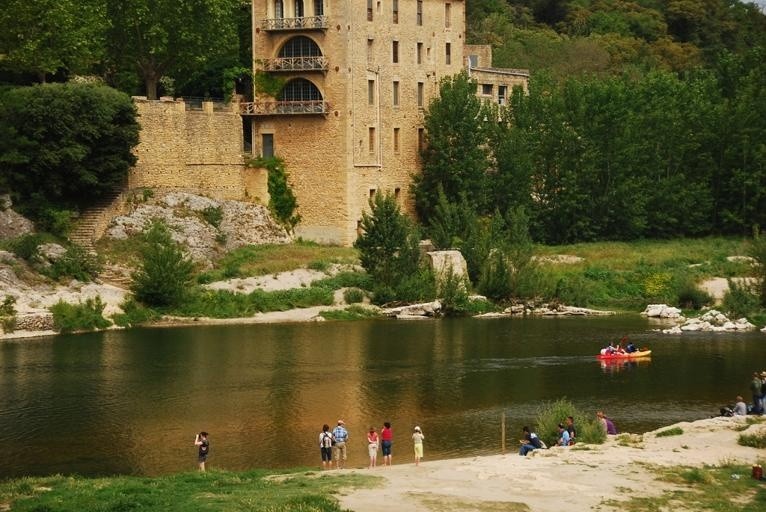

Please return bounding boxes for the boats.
[597,348,652,359]
[599,357,653,369]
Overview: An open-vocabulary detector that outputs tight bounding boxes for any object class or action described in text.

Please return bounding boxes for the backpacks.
[322,432,332,449]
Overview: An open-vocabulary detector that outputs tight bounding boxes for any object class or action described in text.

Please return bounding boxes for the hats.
[760,372,766,377]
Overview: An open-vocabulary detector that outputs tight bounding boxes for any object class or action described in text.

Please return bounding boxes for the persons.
[603,414,616,436]
[193,431,210,471]
[551,422,569,447]
[729,394,746,417]
[596,412,608,437]
[380,421,392,466]
[411,425,425,465]
[565,415,576,443]
[519,425,542,454]
[600,340,648,354]
[318,423,335,469]
[366,425,379,468]
[760,371,766,414]
[751,371,763,414]
[332,419,348,470]
